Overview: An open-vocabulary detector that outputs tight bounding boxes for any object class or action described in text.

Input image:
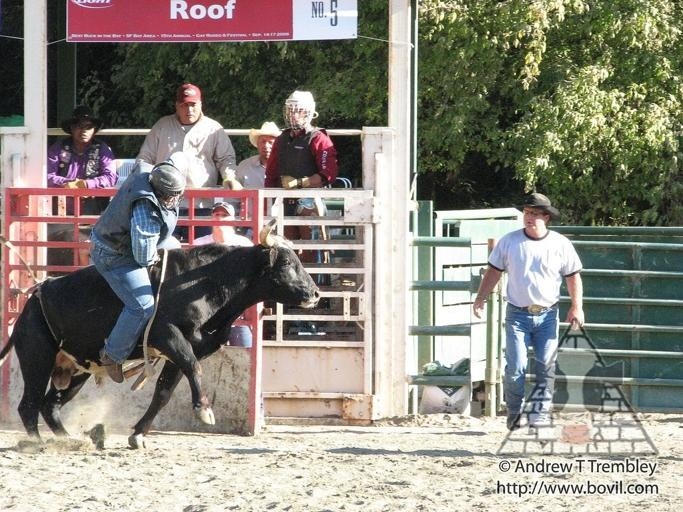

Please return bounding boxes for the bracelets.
[476,293,489,303]
[297,179,302,188]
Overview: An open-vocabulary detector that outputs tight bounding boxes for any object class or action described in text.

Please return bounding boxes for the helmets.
[148,160,187,195]
[285,89,316,120]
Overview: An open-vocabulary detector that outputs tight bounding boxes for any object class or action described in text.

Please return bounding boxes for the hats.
[61,105,102,135]
[210,201,236,217]
[511,192,561,220]
[247,120,283,148]
[176,82,202,106]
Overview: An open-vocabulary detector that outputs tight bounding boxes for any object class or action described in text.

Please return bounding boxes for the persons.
[45,105,118,219]
[233,116,281,240]
[86,151,199,383]
[468,190,586,434]
[261,85,341,340]
[190,200,254,252]
[132,82,245,243]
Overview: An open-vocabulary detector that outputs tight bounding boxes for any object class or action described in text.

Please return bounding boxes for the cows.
[527,349,625,444]
[0,215,322,450]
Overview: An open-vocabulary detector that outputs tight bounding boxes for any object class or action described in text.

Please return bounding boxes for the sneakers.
[527,419,541,436]
[506,413,522,431]
[99,349,125,384]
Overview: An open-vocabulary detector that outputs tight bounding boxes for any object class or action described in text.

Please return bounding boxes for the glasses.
[72,122,97,129]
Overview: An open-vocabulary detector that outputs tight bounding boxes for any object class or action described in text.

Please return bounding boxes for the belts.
[507,301,560,316]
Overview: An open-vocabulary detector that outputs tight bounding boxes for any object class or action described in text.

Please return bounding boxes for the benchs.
[110,159,135,189]
